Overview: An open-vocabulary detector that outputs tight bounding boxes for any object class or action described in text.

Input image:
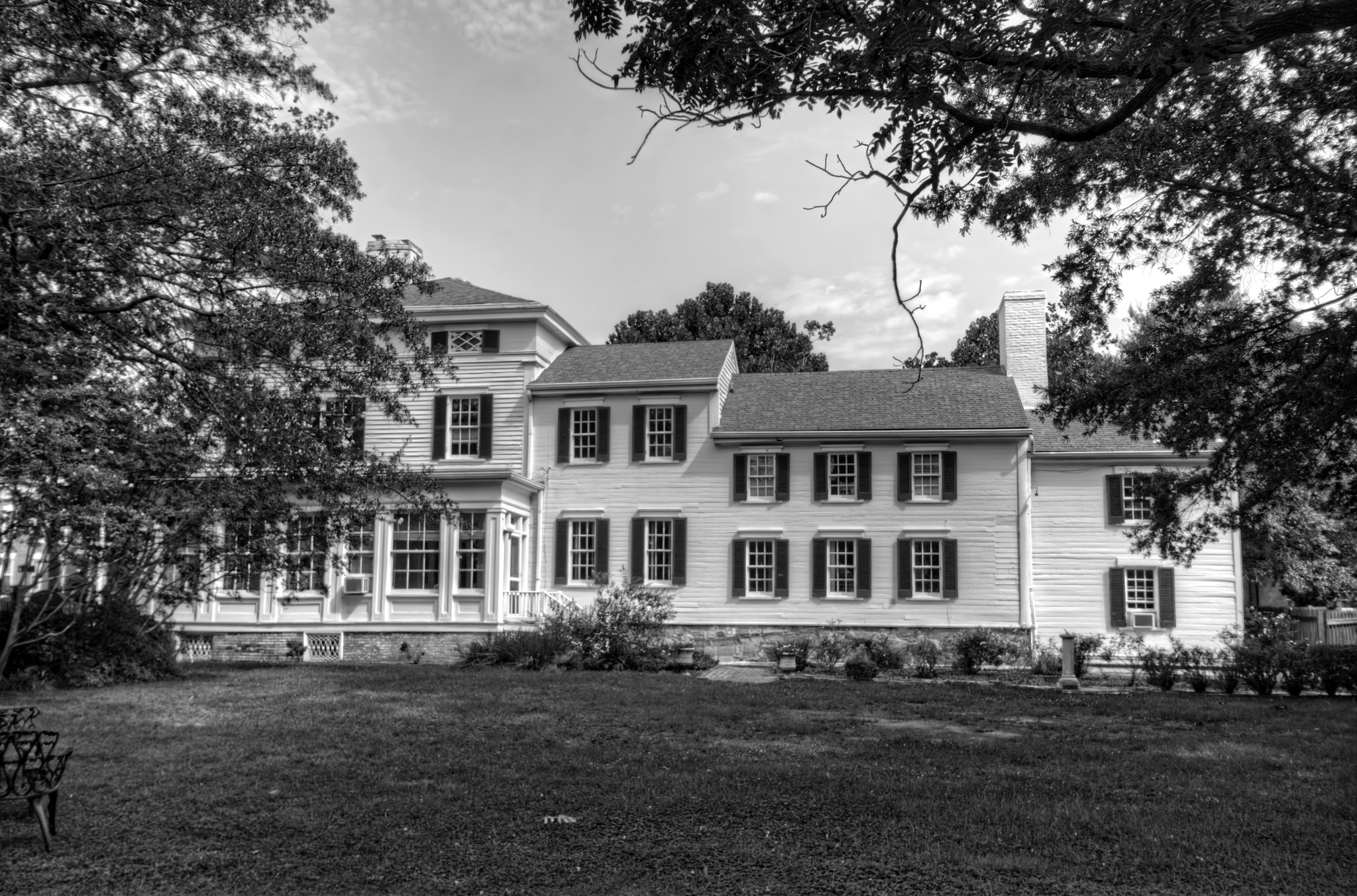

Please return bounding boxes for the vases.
[779,657,796,672]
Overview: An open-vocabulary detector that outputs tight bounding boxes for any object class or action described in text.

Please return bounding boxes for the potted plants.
[672,641,696,665]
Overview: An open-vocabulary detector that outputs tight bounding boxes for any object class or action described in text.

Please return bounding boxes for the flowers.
[776,644,804,657]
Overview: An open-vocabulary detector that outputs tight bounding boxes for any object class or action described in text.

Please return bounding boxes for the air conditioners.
[1130,612,1155,629]
[343,577,369,594]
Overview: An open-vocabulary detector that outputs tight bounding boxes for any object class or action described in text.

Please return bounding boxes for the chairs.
[0,706,73,852]
